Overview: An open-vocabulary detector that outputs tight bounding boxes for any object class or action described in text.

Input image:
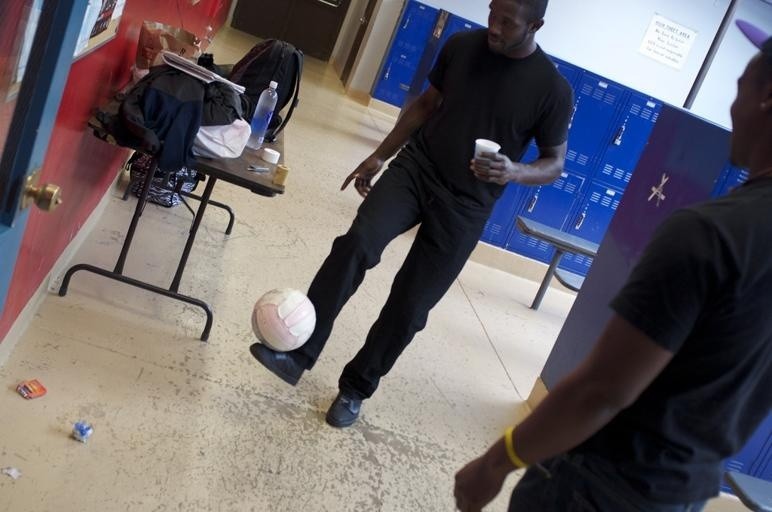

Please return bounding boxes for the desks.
[62,76,288,343]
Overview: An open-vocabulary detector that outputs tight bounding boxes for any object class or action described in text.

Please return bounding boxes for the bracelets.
[504,426,531,469]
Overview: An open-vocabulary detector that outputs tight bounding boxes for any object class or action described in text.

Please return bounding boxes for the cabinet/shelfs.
[365,0,771,495]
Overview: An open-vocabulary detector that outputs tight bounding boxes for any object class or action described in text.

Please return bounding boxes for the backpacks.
[225,37,306,143]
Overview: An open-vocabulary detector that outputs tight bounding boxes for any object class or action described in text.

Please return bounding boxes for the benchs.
[721,468,772,512]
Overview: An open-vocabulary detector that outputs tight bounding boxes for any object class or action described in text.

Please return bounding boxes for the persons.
[453,35,771,511]
[249,0,575,429]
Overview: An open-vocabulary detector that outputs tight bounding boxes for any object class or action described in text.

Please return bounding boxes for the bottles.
[246,81,279,151]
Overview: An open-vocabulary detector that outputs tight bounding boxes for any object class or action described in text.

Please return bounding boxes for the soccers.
[251,288,316,351]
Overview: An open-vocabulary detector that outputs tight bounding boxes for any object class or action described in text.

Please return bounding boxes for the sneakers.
[324,386,363,430]
[246,341,306,390]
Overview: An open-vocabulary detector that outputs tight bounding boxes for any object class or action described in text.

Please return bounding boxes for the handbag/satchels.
[124,145,208,209]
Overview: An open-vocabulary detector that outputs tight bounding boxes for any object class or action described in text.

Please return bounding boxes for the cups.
[261,147,281,164]
[472,138,501,169]
